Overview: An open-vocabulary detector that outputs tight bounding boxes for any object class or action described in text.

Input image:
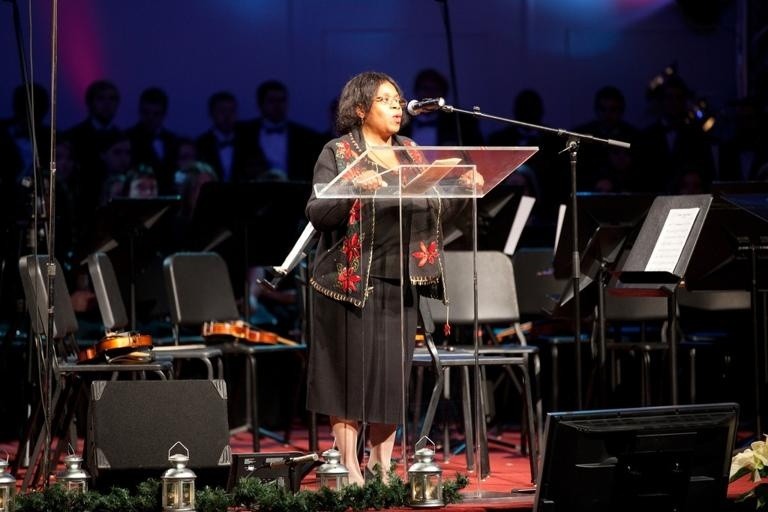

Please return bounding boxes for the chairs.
[86,251,226,385]
[393,250,545,489]
[162,248,305,433]
[546,251,759,407]
[16,250,176,492]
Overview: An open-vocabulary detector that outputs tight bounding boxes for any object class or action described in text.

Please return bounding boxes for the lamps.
[407,436,445,507]
[0,449,17,512]
[160,440,198,512]
[56,443,91,492]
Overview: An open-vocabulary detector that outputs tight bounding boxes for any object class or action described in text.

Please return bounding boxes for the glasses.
[371,93,408,108]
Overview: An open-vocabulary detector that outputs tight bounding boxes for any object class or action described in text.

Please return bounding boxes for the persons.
[1,69,765,351]
[304,71,484,489]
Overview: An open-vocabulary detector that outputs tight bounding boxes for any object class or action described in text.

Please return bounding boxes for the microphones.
[407,97,445,116]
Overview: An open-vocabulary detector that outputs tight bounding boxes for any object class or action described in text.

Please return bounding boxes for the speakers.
[81,378,233,495]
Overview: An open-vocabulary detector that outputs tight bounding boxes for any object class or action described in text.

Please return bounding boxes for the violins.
[203,320,299,346]
[73,329,152,364]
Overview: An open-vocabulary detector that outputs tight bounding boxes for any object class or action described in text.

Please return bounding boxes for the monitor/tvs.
[533,403,739,511]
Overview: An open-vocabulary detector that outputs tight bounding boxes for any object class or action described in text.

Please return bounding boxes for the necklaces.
[365,143,399,174]
